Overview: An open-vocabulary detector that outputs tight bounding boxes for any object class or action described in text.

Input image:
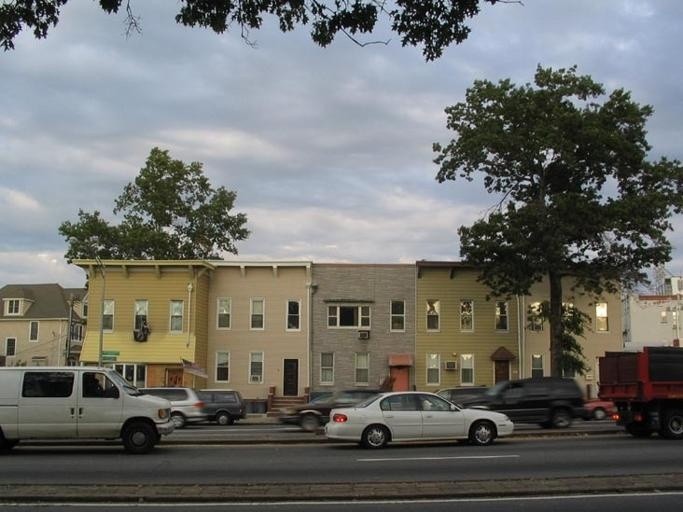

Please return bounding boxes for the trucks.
[597,346,683,439]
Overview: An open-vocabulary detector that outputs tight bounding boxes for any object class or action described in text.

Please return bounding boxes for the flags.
[182,359,209,379]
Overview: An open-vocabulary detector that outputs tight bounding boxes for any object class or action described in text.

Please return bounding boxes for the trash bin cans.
[244,399,266,413]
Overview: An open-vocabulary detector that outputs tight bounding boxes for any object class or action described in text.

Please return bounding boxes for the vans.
[0,366,176,455]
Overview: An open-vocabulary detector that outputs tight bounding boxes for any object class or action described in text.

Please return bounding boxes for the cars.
[138,386,245,430]
